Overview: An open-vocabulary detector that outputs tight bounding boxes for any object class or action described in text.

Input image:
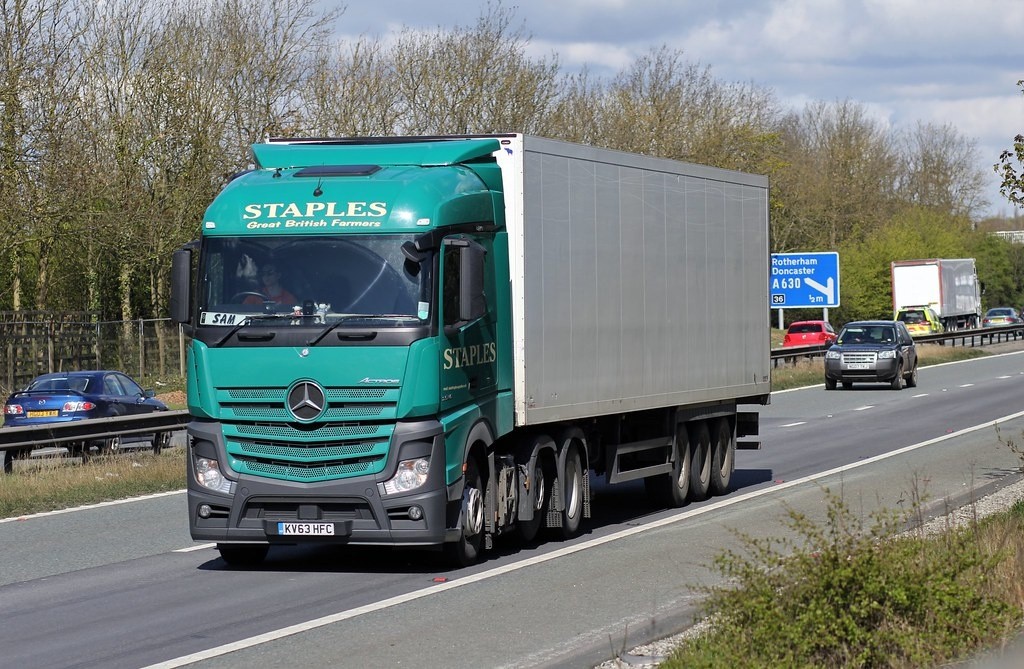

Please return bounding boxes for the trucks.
[891,258,986,332]
[169,135,774,555]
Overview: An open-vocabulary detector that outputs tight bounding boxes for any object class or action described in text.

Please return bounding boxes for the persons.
[242,257,297,305]
[881,329,893,341]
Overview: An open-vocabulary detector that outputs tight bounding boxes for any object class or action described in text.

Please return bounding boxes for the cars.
[2,371,173,460]
[782,321,838,363]
[982,308,1024,336]
[893,308,946,345]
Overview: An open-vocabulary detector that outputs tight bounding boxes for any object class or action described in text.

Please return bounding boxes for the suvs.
[824,321,919,390]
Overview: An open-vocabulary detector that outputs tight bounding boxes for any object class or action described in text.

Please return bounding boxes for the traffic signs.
[770,253,840,308]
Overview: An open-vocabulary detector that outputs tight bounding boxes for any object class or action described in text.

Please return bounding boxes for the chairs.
[55,381,70,393]
